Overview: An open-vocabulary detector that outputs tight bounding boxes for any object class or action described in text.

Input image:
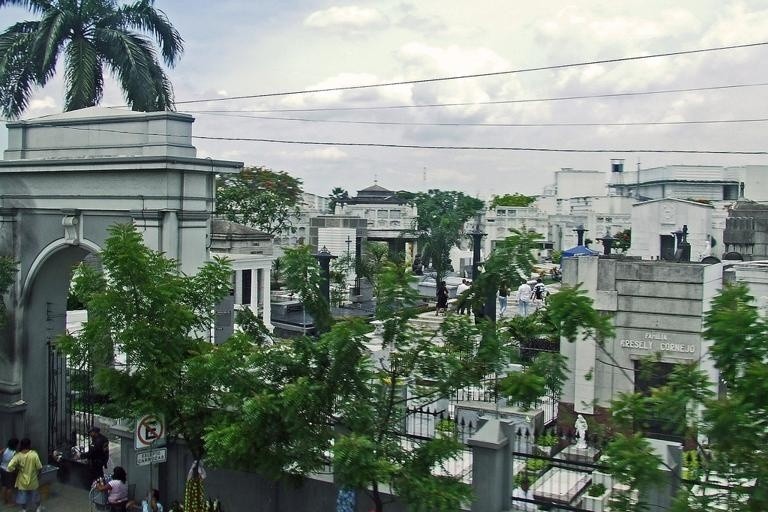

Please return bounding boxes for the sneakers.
[21,506,46,512]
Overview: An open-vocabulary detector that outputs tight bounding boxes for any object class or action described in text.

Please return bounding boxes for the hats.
[87,427,100,433]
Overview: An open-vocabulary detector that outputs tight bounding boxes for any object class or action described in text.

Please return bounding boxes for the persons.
[573,415,587,449]
[456,279,467,315]
[95,466,128,512]
[0,437,19,509]
[433,280,448,316]
[465,281,472,316]
[515,279,531,317]
[7,438,47,512]
[498,284,510,317]
[79,426,110,491]
[529,278,545,310]
[141,489,164,511]
